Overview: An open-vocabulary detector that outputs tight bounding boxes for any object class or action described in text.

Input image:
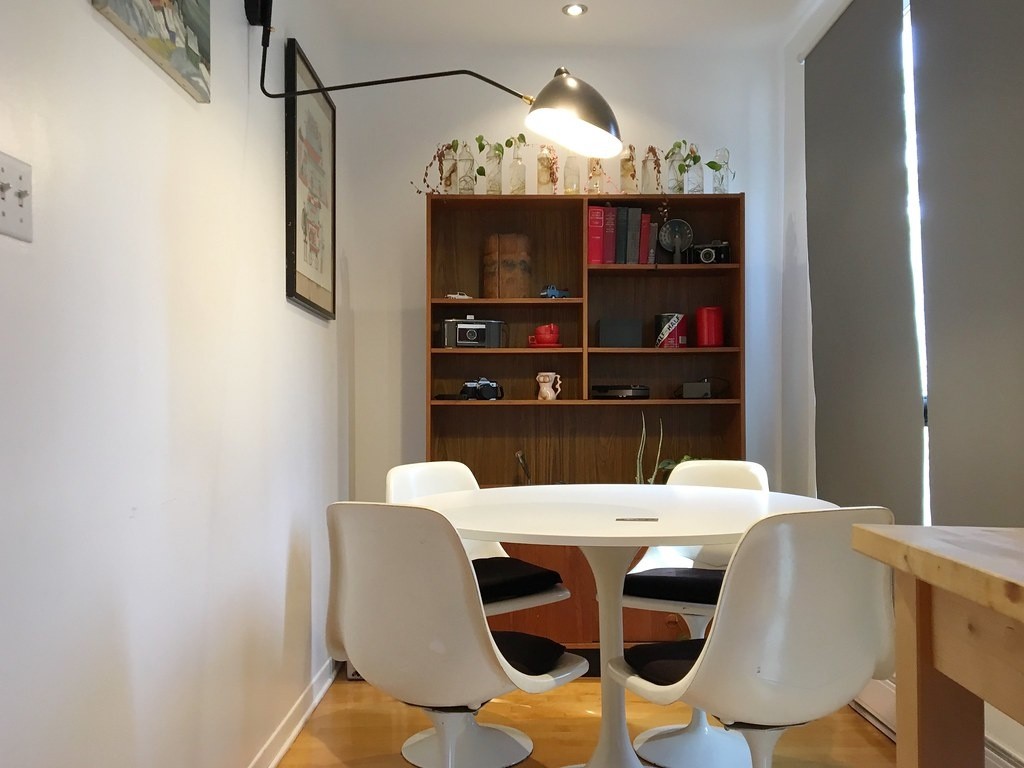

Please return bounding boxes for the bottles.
[458,141,475,195]
[440,143,459,195]
[485,143,504,195]
[686,143,705,194]
[640,145,660,194]
[666,141,685,195]
[509,143,526,194]
[536,138,556,195]
[586,152,604,196]
[712,146,731,194]
[563,148,581,195]
[619,143,638,193]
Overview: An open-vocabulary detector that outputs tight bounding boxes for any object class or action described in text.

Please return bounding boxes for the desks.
[400,484,841,768]
[851,523,1023,768]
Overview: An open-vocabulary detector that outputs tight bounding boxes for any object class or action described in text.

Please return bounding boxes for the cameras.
[441,319,509,348]
[689,240,731,264]
[462,376,504,400]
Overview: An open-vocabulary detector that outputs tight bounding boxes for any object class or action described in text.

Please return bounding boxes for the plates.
[529,341,562,348]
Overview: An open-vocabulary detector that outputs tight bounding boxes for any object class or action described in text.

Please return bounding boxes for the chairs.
[596,460,770,768]
[385,460,571,618]
[325,501,589,768]
[606,505,897,768]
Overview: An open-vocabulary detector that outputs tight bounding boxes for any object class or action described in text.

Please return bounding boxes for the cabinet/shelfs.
[426,192,746,650]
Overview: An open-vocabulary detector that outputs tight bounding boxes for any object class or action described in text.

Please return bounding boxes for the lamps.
[244,0,623,160]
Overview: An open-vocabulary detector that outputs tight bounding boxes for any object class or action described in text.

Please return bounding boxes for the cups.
[693,303,725,348]
[653,311,689,349]
[535,323,559,344]
[536,371,562,400]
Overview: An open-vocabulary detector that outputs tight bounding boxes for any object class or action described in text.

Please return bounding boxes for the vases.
[678,143,705,194]
[505,133,527,196]
[641,145,670,223]
[475,135,504,196]
[452,140,474,196]
[536,144,559,195]
[583,158,624,194]
[705,145,735,194]
[663,141,686,195]
[620,143,638,195]
[409,143,459,195]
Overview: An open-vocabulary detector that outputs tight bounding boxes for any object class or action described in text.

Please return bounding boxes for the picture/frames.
[285,38,336,322]
[91,0,212,104]
